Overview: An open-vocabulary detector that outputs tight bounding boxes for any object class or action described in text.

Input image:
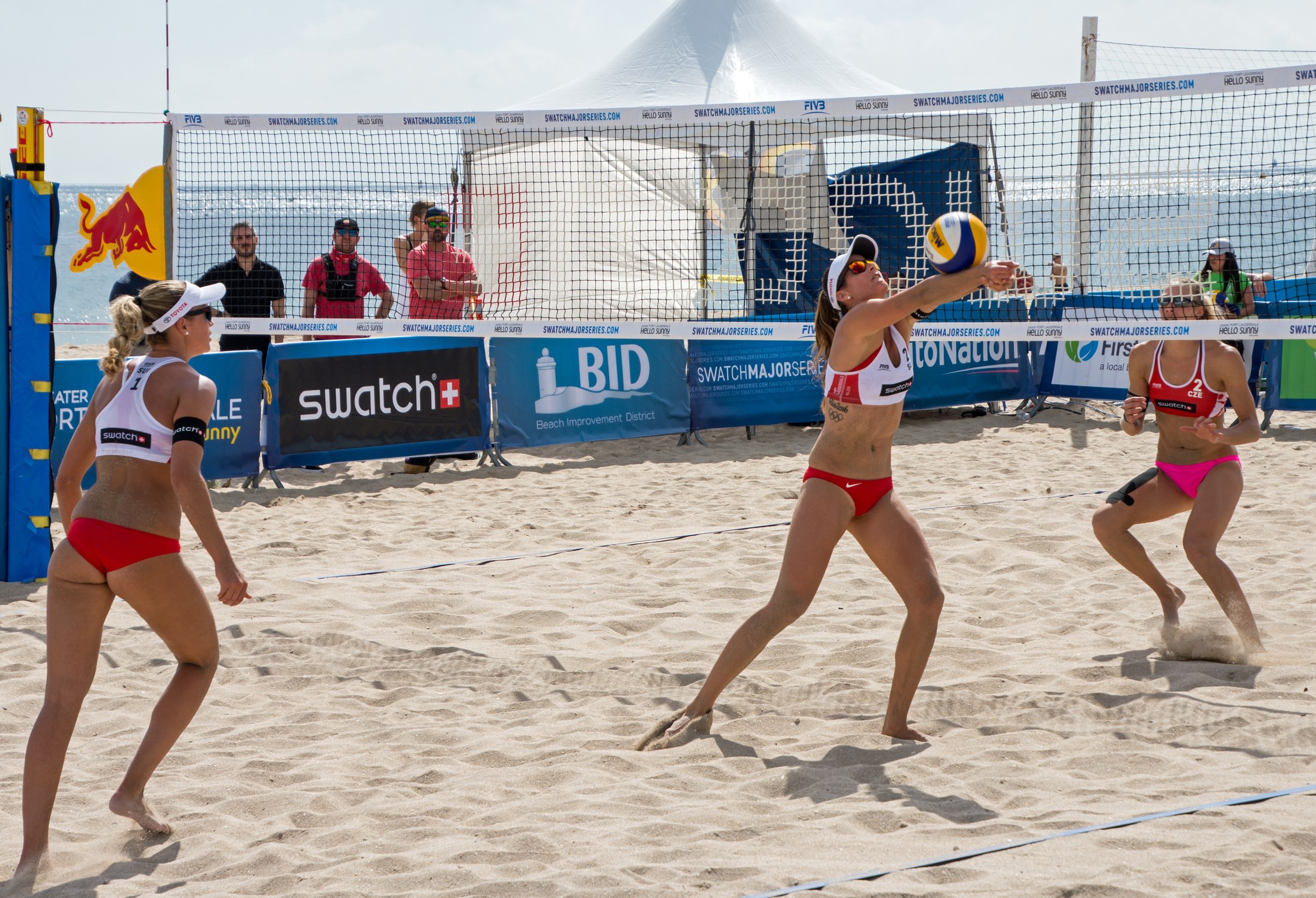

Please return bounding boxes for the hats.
[827,234,879,310]
[333,218,359,231]
[1202,238,1235,255]
[142,281,226,334]
[424,206,451,221]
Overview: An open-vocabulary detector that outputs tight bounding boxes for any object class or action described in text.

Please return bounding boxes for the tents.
[457,0,1017,442]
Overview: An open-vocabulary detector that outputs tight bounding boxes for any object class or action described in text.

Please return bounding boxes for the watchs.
[439,276,446,288]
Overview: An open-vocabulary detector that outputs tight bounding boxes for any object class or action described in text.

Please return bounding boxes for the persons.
[11,280,253,879]
[109,269,160,304]
[392,198,483,473]
[1047,253,1069,292]
[300,218,394,343]
[664,235,1020,741]
[1005,268,1033,294]
[1090,239,1285,655]
[192,222,326,474]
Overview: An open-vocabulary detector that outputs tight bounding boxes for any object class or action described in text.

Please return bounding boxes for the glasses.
[1157,295,1202,307]
[425,221,449,228]
[185,304,213,321]
[836,259,881,291]
[337,227,357,236]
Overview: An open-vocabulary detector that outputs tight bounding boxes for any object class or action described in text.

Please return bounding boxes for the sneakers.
[285,466,323,473]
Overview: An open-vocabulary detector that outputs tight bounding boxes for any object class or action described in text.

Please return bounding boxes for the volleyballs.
[923,211,990,274]
